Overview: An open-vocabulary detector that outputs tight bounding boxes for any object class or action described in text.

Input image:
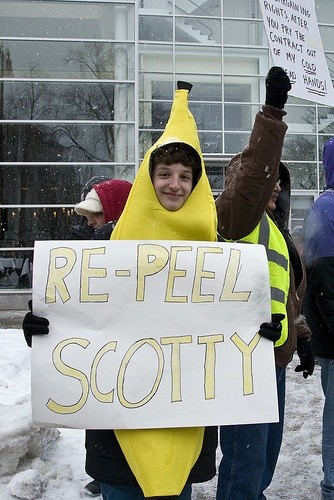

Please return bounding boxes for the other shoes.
[84,479,102,497]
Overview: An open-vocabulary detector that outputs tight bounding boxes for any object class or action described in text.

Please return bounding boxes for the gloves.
[22,300,49,348]
[258,313,285,346]
[294,336,315,379]
[265,66,291,109]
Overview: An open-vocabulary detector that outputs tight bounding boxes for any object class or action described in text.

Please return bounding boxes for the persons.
[301,139,334,499]
[22,67,314,500]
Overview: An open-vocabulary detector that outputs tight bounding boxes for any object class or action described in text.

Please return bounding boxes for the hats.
[75,188,103,216]
